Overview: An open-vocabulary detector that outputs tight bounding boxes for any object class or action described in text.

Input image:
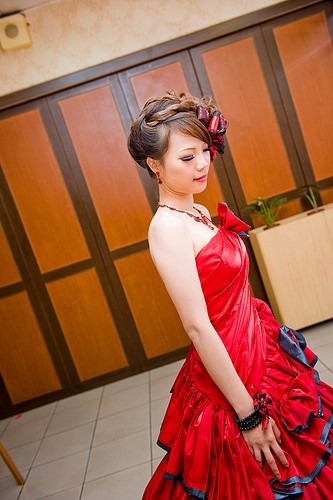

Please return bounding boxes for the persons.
[127,90,333,500]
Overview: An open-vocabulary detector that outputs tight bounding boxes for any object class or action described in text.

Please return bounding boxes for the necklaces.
[157,201,214,230]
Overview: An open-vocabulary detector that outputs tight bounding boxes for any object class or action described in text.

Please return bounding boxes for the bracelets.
[234,391,273,432]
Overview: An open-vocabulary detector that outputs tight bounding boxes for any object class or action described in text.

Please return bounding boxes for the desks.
[248,203,333,331]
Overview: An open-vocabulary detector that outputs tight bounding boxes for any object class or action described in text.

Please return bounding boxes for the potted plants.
[304,187,325,215]
[245,196,288,230]
